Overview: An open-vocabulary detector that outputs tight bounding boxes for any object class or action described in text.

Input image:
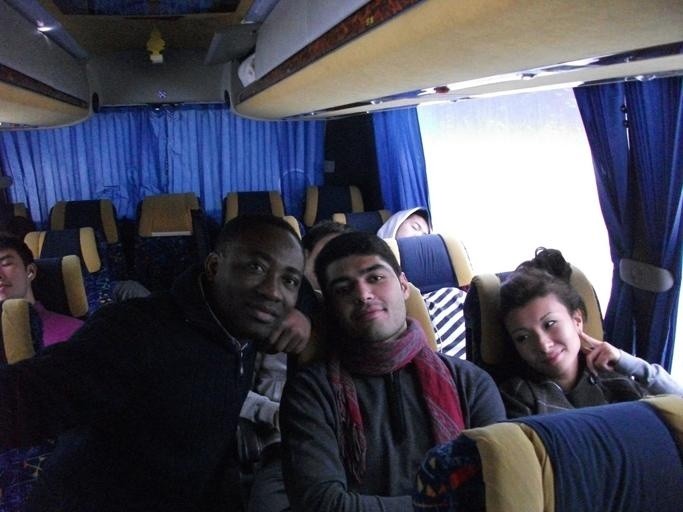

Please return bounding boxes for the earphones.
[27,272,34,279]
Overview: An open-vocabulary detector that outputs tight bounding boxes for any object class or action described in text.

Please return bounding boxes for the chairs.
[222,185,390,235]
[411,395,683,511]
[383,234,603,369]
[0,185,209,367]
